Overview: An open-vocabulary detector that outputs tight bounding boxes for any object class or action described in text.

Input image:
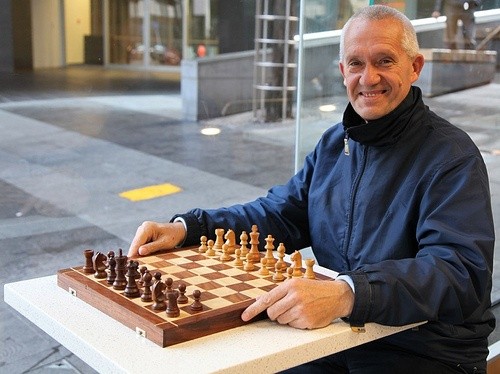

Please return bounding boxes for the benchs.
[412,48,498,96]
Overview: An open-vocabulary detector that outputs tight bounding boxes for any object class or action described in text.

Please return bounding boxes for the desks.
[3,252,428,374]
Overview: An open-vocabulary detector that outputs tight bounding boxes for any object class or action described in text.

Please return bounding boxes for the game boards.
[57,243,336,349]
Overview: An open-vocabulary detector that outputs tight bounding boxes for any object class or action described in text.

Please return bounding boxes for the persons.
[432,0,483,49]
[127,5,496,374]
